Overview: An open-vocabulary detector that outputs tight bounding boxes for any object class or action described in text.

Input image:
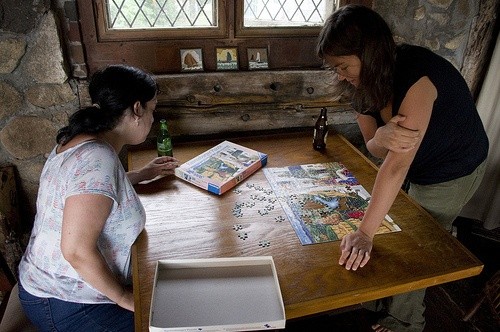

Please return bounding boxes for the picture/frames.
[247,47,269,70]
[179,47,206,73]
[215,46,240,72]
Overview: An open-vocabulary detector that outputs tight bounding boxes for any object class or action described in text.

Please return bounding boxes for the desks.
[127,129,485,332]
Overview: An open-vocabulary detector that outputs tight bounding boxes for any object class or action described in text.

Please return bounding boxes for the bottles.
[157,118,174,171]
[312,108,329,152]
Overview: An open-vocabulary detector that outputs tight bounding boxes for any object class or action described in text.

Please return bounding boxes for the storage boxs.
[175,140,268,195]
[148,255,286,332]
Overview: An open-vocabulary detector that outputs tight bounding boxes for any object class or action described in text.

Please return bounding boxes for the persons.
[315,4,489,332]
[20,64,178,332]
